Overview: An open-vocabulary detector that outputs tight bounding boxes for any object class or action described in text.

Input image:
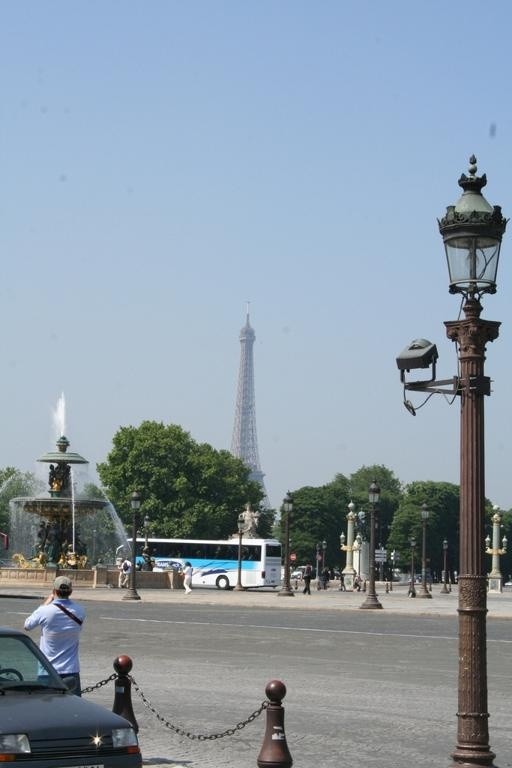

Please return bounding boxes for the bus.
[116,537,282,590]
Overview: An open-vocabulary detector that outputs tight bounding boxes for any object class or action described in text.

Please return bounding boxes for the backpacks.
[122,561,128,571]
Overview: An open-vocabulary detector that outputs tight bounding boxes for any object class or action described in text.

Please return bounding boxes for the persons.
[181,562,193,594]
[49,463,54,488]
[355,577,363,591]
[28,519,87,561]
[341,575,345,591]
[303,563,312,594]
[63,465,71,482]
[320,566,330,581]
[118,560,124,587]
[23,575,87,697]
[122,556,131,588]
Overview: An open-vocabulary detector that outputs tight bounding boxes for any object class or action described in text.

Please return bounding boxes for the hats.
[54,576,73,593]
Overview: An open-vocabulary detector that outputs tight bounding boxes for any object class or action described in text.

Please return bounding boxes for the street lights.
[278,480,450,609]
[122,489,141,600]
[232,514,246,591]
[436,156,511,768]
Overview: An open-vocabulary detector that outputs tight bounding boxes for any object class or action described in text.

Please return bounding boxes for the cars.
[0,627,142,768]
